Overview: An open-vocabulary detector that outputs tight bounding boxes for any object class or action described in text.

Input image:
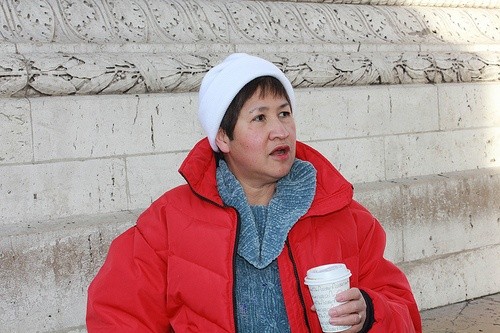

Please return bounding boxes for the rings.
[357,313,363,325]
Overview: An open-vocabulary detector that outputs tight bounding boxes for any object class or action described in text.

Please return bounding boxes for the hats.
[196,53,296,152]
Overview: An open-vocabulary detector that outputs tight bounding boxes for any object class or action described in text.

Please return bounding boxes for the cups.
[303,263,355,333]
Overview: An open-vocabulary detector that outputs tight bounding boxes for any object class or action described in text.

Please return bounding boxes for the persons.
[85,52,422,333]
